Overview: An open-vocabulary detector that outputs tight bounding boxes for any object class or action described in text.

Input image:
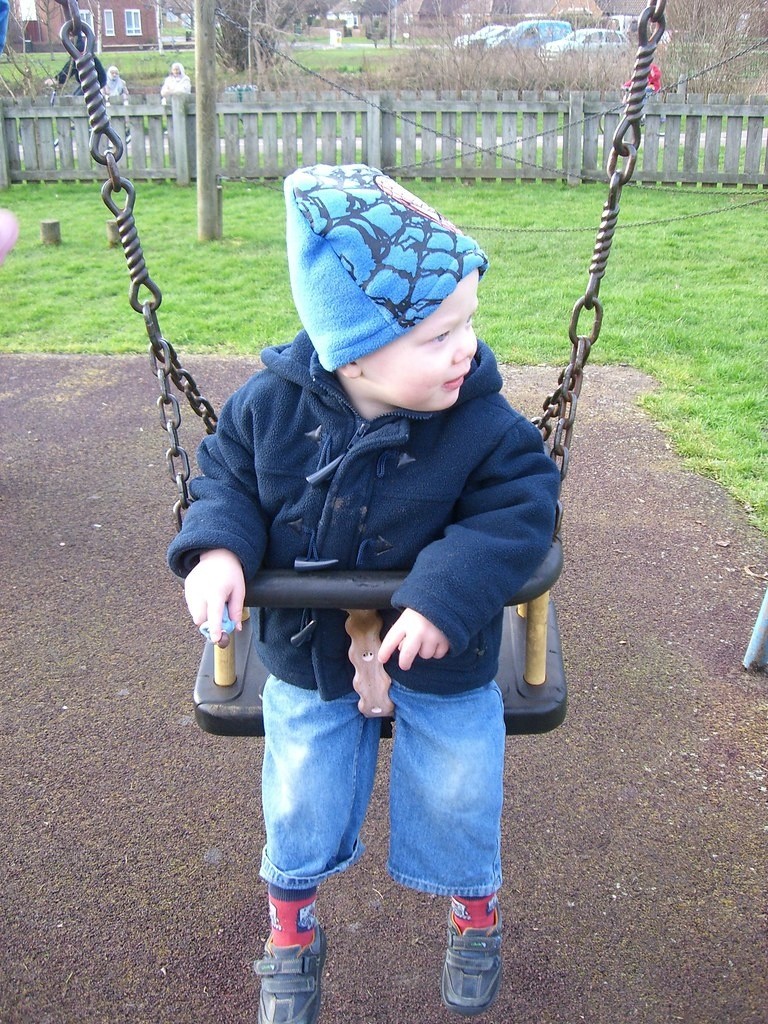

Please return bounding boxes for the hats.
[282,164,490,373]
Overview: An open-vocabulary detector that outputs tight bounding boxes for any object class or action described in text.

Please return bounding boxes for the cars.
[547,28,633,65]
[454,25,511,51]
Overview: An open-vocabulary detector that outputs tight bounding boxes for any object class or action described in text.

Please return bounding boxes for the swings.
[60,0,673,742]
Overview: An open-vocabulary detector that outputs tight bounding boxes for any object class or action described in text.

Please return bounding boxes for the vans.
[486,19,571,51]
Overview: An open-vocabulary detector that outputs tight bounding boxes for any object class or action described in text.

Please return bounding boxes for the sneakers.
[253,923,326,1024]
[442,895,502,1016]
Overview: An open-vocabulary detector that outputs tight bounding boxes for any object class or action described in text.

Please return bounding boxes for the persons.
[624,62,662,120]
[160,62,191,106]
[44,30,108,100]
[99,65,130,103]
[165,164,560,1024]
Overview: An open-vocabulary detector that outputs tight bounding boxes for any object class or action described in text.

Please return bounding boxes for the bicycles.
[599,87,668,138]
[18,82,136,148]
